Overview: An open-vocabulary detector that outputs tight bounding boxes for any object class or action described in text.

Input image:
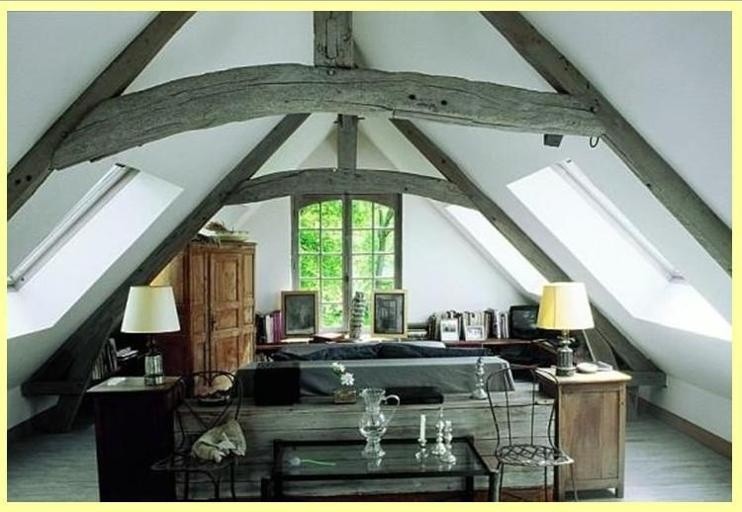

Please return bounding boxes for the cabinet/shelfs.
[81,375,181,502]
[532,366,634,502]
[149,241,258,397]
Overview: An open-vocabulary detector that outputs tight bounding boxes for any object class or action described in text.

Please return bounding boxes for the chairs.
[487,365,581,502]
[150,368,244,502]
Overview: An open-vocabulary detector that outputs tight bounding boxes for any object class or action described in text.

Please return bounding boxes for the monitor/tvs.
[508,305,547,341]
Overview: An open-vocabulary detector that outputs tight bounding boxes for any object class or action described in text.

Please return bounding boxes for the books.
[254,314,273,343]
[308,332,344,343]
[92,338,118,380]
[429,308,509,339]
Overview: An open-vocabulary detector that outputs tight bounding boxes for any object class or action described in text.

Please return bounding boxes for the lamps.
[120,285,181,386]
[535,280,595,376]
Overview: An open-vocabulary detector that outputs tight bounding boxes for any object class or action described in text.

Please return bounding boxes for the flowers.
[328,360,354,391]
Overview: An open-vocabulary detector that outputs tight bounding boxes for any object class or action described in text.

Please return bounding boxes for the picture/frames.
[438,318,486,342]
[281,289,321,338]
[371,290,407,337]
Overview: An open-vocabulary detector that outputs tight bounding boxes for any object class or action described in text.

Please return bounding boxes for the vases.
[333,388,356,403]
